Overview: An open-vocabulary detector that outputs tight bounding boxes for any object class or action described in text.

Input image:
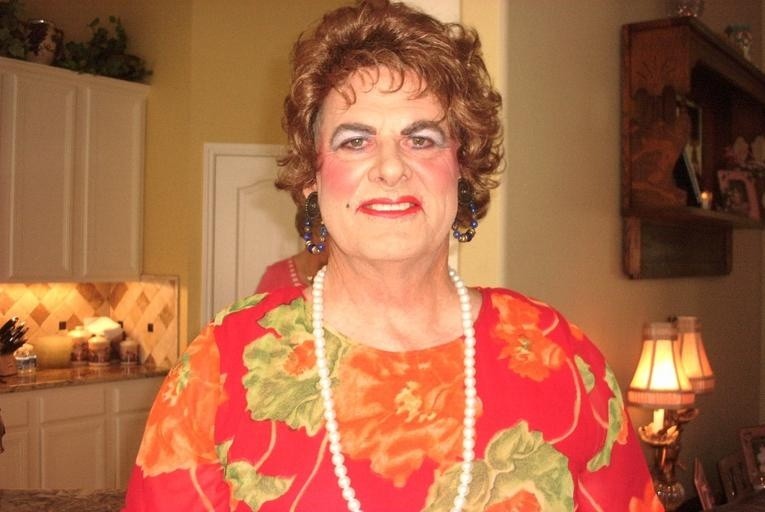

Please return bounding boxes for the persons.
[121,1,666,512]
[255,200,329,293]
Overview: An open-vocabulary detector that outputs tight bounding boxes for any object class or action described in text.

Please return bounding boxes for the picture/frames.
[738,425,765,493]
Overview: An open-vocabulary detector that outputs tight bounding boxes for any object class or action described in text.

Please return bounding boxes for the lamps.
[627,315,716,509]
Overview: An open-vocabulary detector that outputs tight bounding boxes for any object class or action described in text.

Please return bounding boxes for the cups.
[14,355,39,379]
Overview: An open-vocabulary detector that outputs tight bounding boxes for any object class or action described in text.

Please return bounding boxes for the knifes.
[0,315,31,355]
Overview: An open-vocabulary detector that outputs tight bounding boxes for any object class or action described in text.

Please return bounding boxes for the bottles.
[86,332,112,367]
[57,320,68,336]
[119,337,138,366]
[67,325,92,363]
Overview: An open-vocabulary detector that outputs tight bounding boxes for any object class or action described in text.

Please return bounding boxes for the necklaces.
[310,264,475,512]
[304,273,313,281]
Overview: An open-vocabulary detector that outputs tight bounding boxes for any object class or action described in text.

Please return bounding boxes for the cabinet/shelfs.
[1,54,152,283]
[0,376,169,491]
[620,14,764,280]
